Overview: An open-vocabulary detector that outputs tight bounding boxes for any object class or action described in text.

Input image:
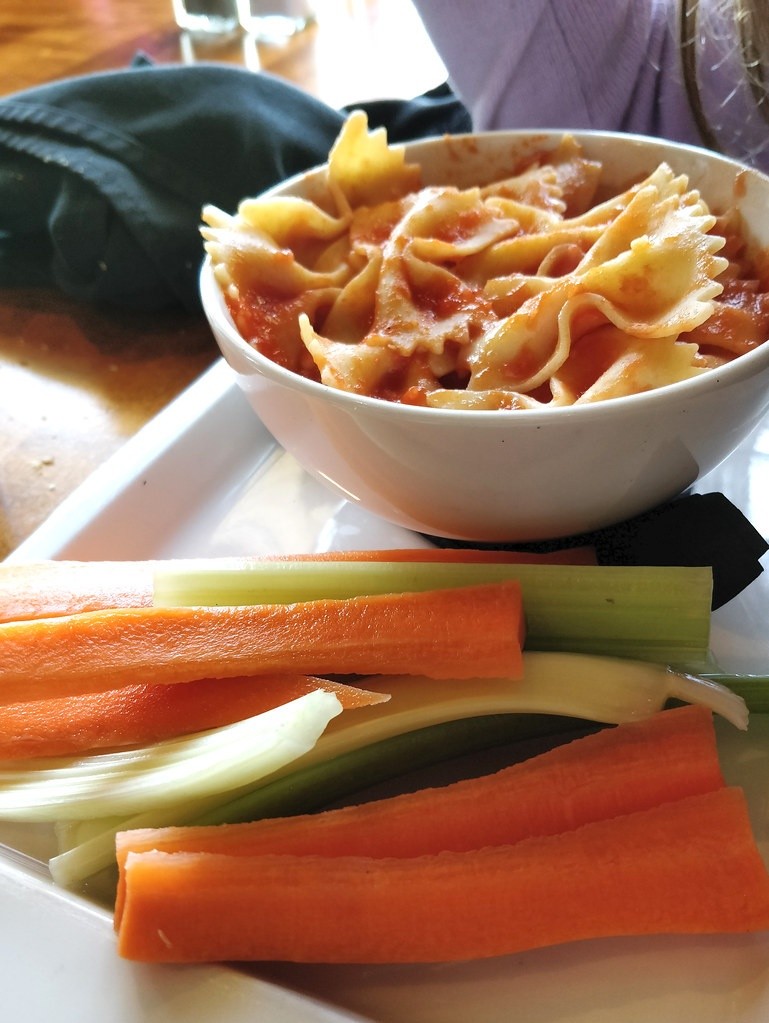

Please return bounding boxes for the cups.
[172,0,318,41]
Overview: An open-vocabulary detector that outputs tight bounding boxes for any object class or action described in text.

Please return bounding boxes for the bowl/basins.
[199,126,769,542]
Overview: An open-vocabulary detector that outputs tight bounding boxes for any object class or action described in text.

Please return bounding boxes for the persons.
[412,0,769,176]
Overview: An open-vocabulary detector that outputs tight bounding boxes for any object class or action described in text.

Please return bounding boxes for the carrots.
[0,548,769,966]
